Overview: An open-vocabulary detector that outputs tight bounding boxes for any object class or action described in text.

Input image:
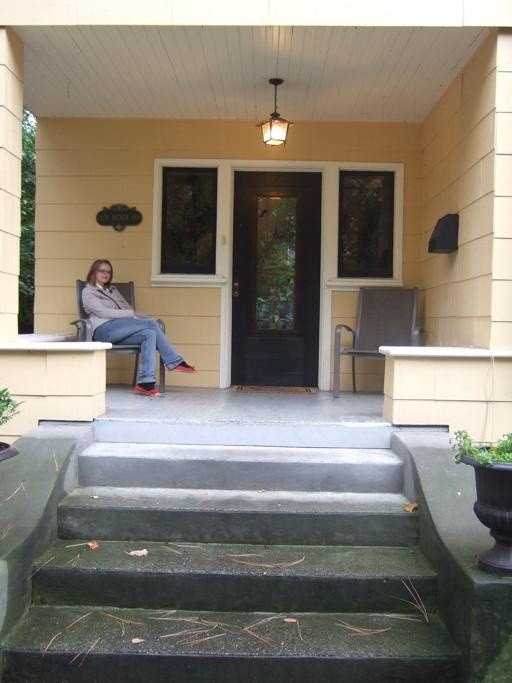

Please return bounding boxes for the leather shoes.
[135,384,159,397]
[173,365,198,373]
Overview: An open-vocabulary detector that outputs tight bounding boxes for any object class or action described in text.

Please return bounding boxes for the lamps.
[254,78,296,148]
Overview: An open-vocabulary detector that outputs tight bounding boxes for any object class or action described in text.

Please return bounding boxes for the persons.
[78,257,197,399]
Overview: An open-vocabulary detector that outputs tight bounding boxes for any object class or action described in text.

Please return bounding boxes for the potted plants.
[448,426,511,574]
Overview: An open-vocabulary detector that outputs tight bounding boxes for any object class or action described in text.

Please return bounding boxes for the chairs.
[68,279,166,393]
[332,285,425,398]
